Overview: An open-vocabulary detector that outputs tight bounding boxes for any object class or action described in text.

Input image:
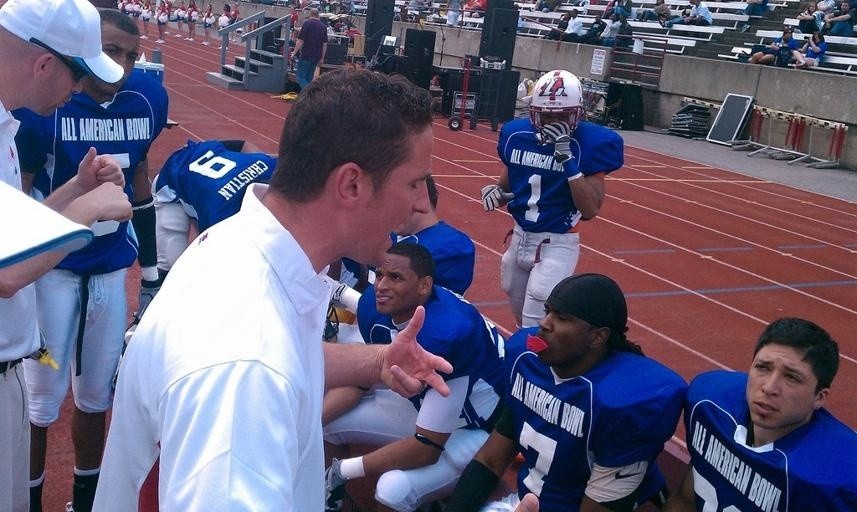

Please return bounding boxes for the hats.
[0,0,125,84]
[546,274,627,331]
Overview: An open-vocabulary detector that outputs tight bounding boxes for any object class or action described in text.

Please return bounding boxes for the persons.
[817,0,835,14]
[218,4,229,50]
[821,1,854,37]
[132,0,143,24]
[173,0,186,38]
[183,0,201,42]
[600,13,620,47]
[561,9,582,42]
[445,0,463,26]
[663,317,857,512]
[465,0,487,18]
[614,0,632,17]
[229,2,239,42]
[581,15,606,45]
[320,244,507,512]
[659,0,696,28]
[545,14,564,39]
[685,0,712,26]
[797,1,822,34]
[12,7,169,512]
[639,0,670,22]
[327,177,476,346]
[748,28,792,63]
[1,0,133,511]
[615,15,632,48]
[291,7,328,89]
[90,70,435,512]
[164,0,172,35]
[120,0,125,13]
[201,4,213,46]
[535,0,562,13]
[140,0,151,39]
[155,1,166,44]
[447,272,688,512]
[548,10,571,39]
[289,7,298,39]
[736,0,768,33]
[793,30,826,70]
[151,139,276,285]
[480,74,624,330]
[601,0,617,18]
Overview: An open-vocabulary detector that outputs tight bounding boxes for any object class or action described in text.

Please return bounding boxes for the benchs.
[784,18,857,35]
[744,30,857,56]
[394,1,750,54]
[716,46,857,76]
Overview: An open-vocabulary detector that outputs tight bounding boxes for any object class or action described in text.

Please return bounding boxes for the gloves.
[325,457,347,512]
[541,121,573,163]
[480,184,515,211]
[135,279,161,324]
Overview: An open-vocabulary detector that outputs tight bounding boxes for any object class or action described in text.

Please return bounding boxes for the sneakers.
[226,47,229,51]
[218,46,222,49]
[158,39,165,43]
[204,42,208,46]
[796,60,807,68]
[201,42,205,44]
[659,18,665,27]
[156,39,160,42]
[140,35,148,40]
[184,38,189,40]
[188,38,193,42]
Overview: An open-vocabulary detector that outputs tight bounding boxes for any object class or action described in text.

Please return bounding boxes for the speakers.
[256,17,281,48]
[363,0,396,55]
[403,28,437,91]
[478,7,520,69]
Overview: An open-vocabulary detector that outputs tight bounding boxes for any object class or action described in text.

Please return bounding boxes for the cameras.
[804,35,816,43]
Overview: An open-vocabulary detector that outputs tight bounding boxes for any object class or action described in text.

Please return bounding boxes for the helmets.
[528,69,584,132]
